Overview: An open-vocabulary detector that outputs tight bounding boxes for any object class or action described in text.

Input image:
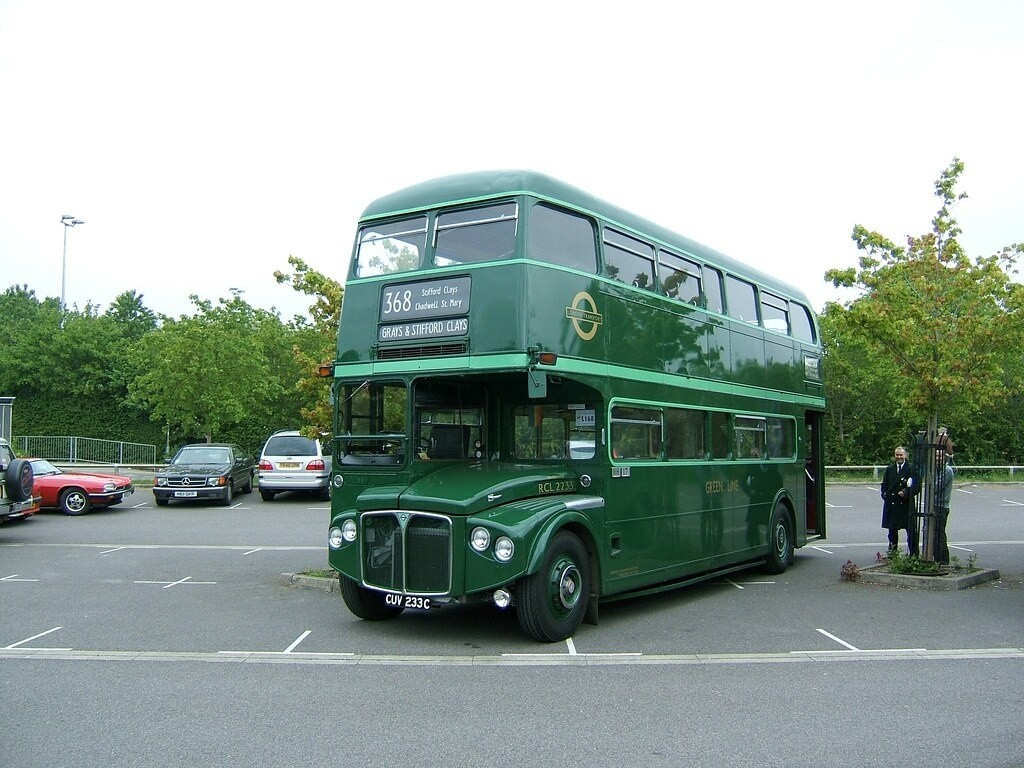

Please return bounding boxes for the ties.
[899,464,902,474]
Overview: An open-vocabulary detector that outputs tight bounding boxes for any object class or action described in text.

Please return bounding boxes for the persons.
[881,446,922,557]
[923,450,954,565]
[910,427,954,476]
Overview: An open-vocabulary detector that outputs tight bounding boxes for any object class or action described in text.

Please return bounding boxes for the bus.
[319,166,828,644]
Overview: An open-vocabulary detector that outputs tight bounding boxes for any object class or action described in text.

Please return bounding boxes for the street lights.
[60,213,85,314]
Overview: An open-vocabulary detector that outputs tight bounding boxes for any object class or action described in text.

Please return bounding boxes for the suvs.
[0,438,36,525]
[258,429,342,501]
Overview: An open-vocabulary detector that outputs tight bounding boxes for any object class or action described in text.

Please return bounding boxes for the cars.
[19,457,135,515]
[152,442,255,506]
